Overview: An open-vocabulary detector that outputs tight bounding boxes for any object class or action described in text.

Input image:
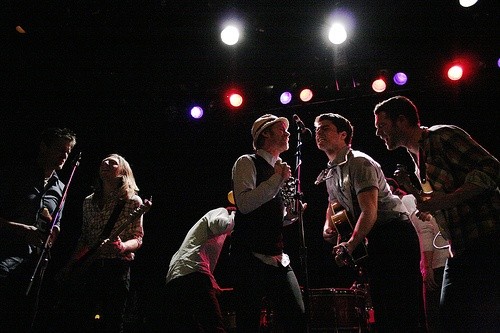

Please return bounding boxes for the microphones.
[293,114,312,138]
[72,152,82,166]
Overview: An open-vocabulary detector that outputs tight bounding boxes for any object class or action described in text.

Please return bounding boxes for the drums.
[309,288,367,330]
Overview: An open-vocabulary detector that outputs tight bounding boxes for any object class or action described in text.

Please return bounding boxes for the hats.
[251,113,289,150]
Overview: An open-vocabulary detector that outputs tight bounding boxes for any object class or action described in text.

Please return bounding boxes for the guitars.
[331,199,368,266]
[72,199,152,277]
[394,164,451,241]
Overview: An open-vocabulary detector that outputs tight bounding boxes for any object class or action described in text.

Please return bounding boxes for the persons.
[74,152,145,333]
[0,126,76,333]
[232,113,307,333]
[373,96,500,333]
[165,206,235,333]
[313,113,426,333]
